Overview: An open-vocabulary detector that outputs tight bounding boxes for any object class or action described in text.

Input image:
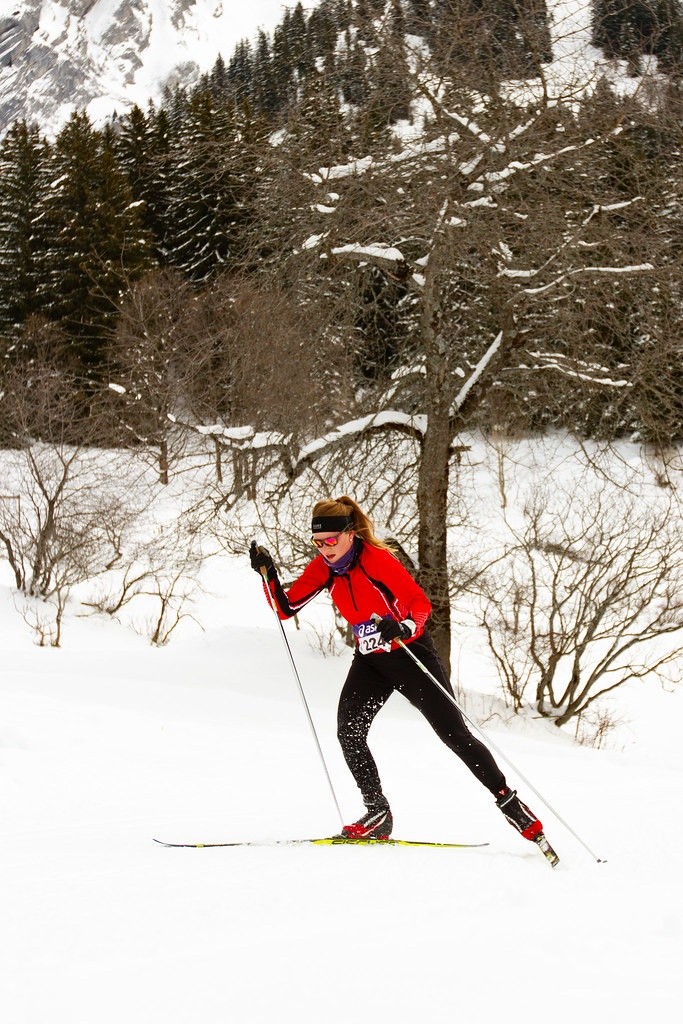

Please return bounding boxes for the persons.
[249,497,543,842]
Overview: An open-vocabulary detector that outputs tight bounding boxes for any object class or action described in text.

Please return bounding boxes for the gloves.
[370,618,404,643]
[249,540,274,576]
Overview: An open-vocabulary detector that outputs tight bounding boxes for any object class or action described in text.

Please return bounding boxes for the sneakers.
[341,806,393,839]
[495,788,543,841]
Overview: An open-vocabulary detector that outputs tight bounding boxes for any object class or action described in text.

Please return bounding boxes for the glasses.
[310,532,343,548]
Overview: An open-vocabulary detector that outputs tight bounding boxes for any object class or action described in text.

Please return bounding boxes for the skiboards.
[151,831,563,868]
